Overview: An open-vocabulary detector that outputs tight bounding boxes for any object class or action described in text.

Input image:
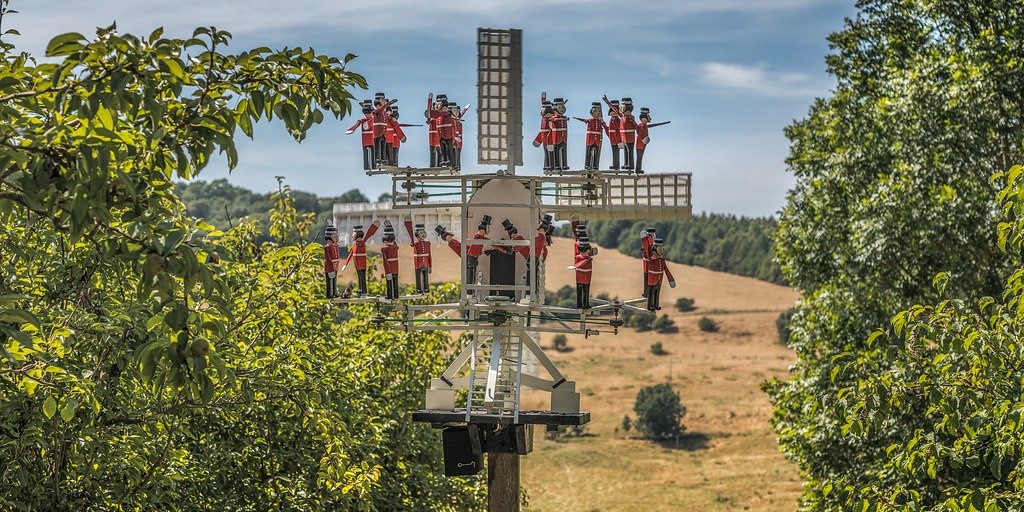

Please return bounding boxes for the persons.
[346,93,471,175]
[324,214,677,311]
[533,91,671,174]
